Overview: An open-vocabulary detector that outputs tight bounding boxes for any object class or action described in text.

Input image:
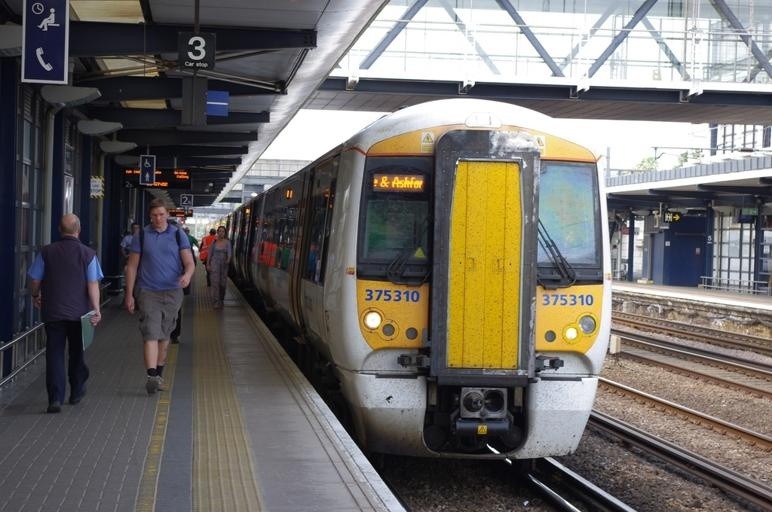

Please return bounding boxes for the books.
[81,309,97,350]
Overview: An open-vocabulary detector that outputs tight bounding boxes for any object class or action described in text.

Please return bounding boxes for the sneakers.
[147,376,164,393]
[171,337,177,343]
[48,401,60,412]
[70,385,86,404]
[214,304,223,309]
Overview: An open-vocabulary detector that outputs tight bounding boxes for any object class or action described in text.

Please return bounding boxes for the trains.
[200,94,617,481]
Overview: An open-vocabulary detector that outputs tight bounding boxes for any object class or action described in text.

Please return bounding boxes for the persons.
[206,226,233,310]
[183,227,200,267]
[120,223,142,311]
[27,214,106,410]
[126,197,197,393]
[166,219,191,344]
[199,228,218,288]
